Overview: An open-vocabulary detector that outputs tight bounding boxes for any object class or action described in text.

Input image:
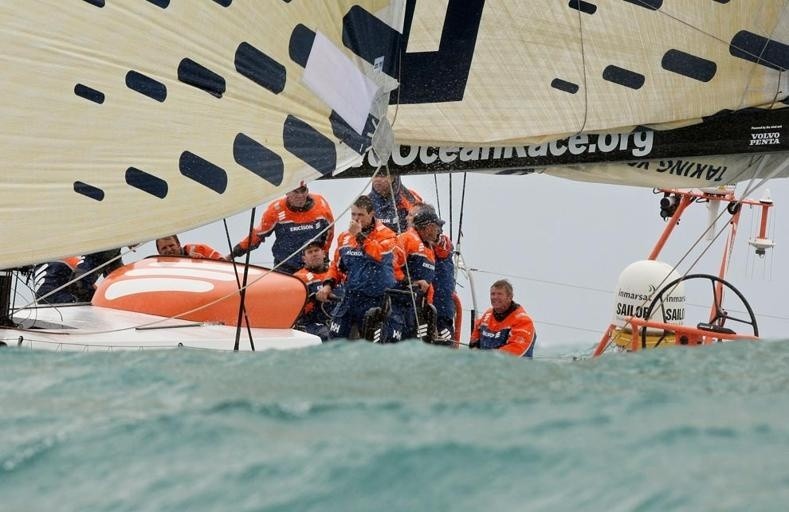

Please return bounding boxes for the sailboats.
[0,0,788,350]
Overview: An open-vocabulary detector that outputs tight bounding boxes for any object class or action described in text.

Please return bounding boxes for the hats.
[302,239,322,247]
[413,209,445,226]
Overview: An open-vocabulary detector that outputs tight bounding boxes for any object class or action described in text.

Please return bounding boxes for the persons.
[32,258,81,303]
[156,234,224,259]
[224,185,334,275]
[404,203,455,346]
[469,280,536,358]
[292,241,339,342]
[369,173,422,233]
[71,243,140,302]
[388,211,447,343]
[315,196,398,344]
